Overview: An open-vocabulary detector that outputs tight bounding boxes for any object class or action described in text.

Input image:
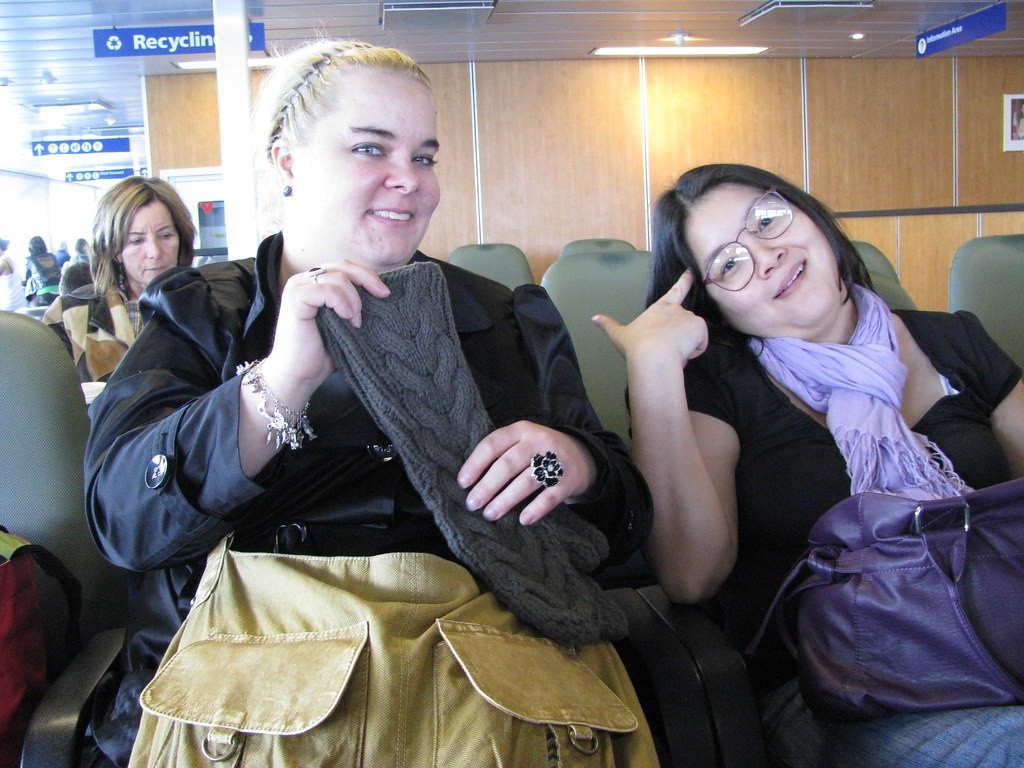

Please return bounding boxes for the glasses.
[701,185,794,291]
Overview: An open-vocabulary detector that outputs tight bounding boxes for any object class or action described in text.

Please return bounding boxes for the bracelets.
[234,360,317,450]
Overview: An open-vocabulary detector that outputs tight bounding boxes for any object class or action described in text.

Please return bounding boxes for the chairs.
[0,233,1024,768]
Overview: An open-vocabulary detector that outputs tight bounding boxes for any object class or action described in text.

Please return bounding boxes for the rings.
[306,265,327,284]
[531,451,564,486]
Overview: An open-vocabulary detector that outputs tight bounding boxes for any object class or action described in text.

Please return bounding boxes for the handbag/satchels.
[749,473,1024,710]
[0,525,83,768]
[122,531,661,768]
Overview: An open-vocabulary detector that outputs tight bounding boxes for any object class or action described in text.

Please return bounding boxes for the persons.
[0,234,89,313]
[592,163,1024,768]
[39,175,196,383]
[82,37,657,768]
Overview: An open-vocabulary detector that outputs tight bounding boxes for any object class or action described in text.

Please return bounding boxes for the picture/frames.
[1003,94,1024,152]
